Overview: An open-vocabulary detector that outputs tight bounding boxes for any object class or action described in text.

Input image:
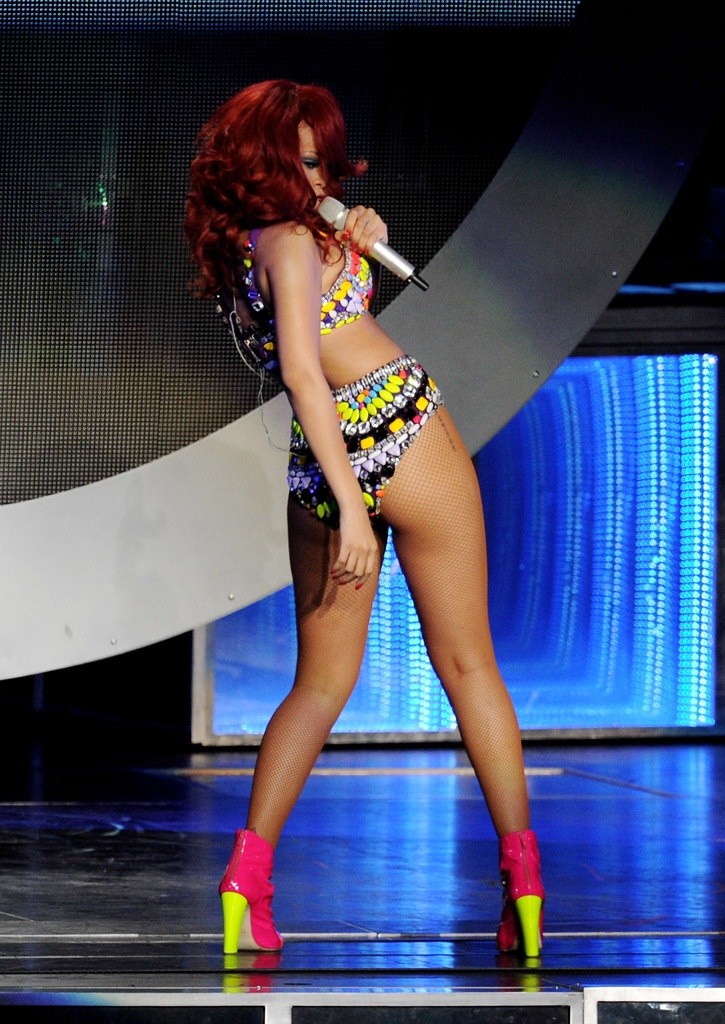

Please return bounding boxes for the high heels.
[497,829,544,957]
[219,829,283,954]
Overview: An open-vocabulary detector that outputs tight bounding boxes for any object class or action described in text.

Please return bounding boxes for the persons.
[183,80,545,956]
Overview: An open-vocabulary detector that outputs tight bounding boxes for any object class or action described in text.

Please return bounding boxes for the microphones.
[318,196,430,292]
